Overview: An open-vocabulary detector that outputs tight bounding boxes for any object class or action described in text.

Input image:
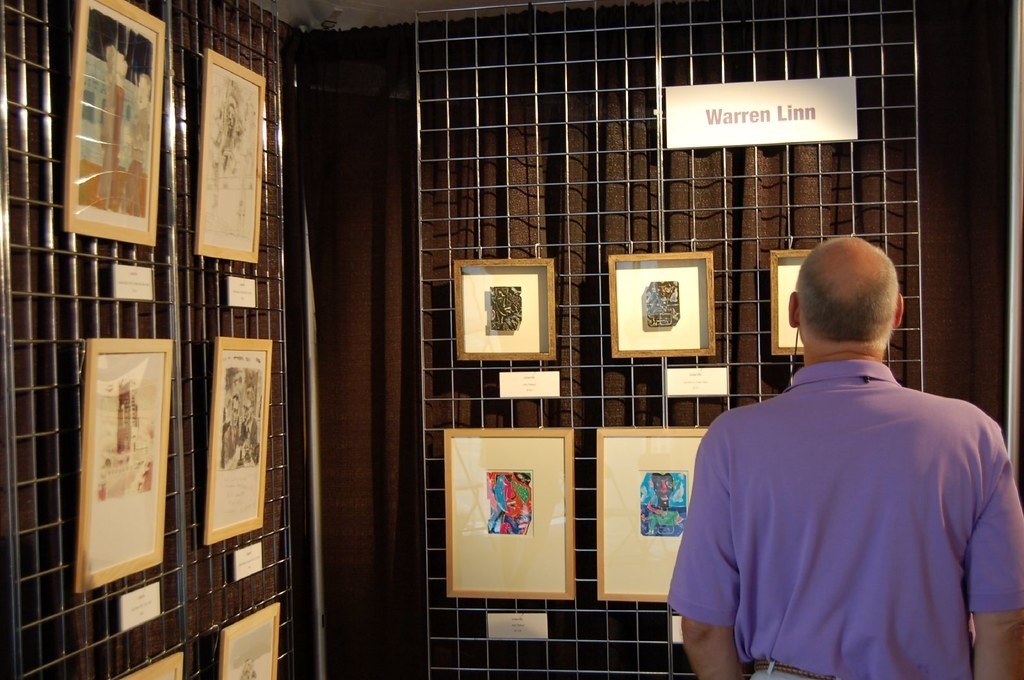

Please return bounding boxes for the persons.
[666,236,1023,680]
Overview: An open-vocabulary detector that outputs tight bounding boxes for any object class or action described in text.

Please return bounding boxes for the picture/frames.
[596,427,709,602]
[608,250,715,358]
[770,250,813,355]
[75,339,174,593]
[219,602,280,680]
[444,428,575,600]
[453,258,556,360]
[63,0,165,247]
[121,652,184,680]
[195,47,266,264]
[204,337,273,545]
